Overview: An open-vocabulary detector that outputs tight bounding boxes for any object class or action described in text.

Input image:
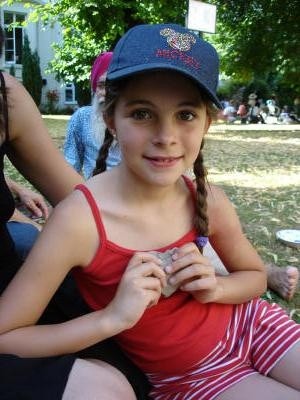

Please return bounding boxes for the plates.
[276,229,300,249]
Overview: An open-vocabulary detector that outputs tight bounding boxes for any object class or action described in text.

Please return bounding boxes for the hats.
[105,23,224,112]
[91,52,114,93]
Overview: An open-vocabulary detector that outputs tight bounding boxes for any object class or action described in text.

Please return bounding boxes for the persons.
[0,67,138,400]
[217,91,296,127]
[0,25,299,399]
[0,174,49,233]
[64,50,125,180]
